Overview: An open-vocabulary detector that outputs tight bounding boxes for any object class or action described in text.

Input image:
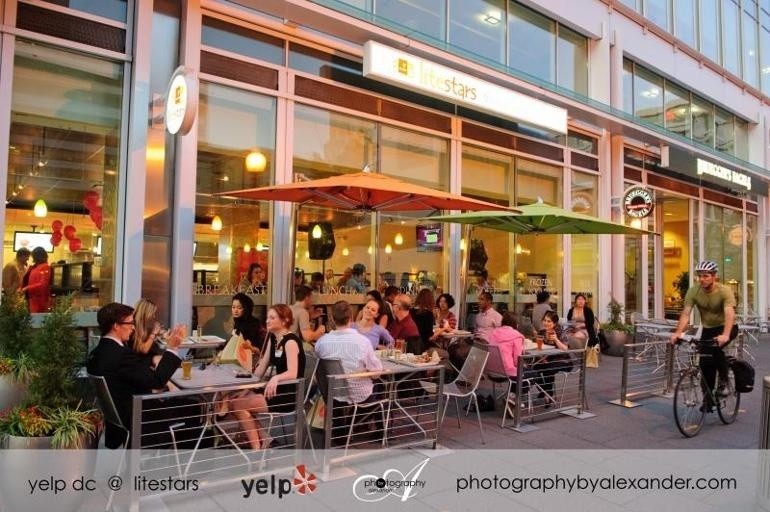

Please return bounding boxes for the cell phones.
[236,374,252,378]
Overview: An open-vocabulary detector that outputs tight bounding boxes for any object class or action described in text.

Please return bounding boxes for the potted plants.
[600,298,632,356]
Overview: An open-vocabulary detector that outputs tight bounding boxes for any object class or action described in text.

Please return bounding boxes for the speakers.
[468,239,488,270]
[308,222,336,259]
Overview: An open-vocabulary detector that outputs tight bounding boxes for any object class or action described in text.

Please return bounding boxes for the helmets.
[695,261,717,276]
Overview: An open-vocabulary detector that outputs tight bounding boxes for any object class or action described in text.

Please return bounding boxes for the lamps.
[211,171,222,231]
[32,124,49,218]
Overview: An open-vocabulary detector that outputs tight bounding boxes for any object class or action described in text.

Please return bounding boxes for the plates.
[455,331,471,335]
[391,355,439,369]
[440,333,453,336]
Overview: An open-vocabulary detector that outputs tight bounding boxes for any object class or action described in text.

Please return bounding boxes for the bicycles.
[672,335,743,438]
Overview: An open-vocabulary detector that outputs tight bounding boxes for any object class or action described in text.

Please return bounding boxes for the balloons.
[50,191,102,252]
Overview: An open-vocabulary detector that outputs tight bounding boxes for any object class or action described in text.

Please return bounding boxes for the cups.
[181,354,194,381]
[394,351,402,360]
[394,337,406,353]
[546,328,554,341]
[536,336,544,351]
[196,324,208,343]
[525,339,532,348]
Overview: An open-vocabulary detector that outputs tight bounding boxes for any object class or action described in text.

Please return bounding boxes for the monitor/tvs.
[416,223,443,252]
[13,231,54,253]
[96,236,102,256]
[193,241,197,258]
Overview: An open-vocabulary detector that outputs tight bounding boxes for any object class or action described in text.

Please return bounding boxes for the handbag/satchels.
[306,395,326,429]
[733,359,755,393]
[584,347,599,369]
[220,332,253,373]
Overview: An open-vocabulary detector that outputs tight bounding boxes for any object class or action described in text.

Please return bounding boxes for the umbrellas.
[417,196,661,330]
[213,164,523,290]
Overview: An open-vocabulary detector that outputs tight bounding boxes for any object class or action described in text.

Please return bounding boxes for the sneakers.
[699,404,712,413]
[715,379,731,397]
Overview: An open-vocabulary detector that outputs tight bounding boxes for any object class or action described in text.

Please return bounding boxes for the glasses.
[118,319,134,326]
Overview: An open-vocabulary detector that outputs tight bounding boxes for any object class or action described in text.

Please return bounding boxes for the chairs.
[84,316,602,512]
[632,308,763,377]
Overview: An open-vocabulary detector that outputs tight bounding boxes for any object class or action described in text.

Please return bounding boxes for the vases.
[0,428,100,511]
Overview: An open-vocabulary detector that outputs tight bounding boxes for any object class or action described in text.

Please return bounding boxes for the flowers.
[0,288,107,453]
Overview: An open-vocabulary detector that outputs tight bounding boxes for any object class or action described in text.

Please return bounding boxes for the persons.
[19,246,51,313]
[670,261,739,412]
[2,247,31,295]
